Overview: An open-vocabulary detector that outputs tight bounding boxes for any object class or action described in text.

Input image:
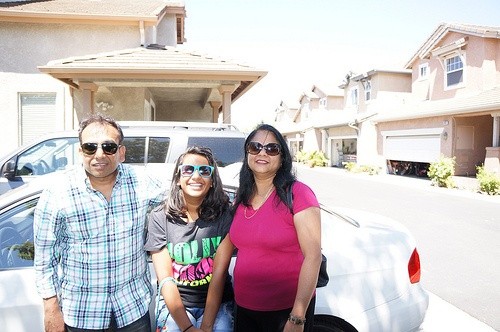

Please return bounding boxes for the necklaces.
[187,211,199,221]
[244,183,275,219]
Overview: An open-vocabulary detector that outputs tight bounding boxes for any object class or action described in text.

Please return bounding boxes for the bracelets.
[183,324,193,332]
[288,313,306,325]
[43,295,57,300]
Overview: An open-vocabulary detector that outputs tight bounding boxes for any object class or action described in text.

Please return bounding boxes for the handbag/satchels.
[288,181,329,288]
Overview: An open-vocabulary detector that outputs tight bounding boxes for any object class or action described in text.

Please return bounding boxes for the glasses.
[178,164,214,177]
[246,142,283,156]
[81,143,122,155]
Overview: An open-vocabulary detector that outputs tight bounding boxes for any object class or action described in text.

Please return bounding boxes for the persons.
[142,145,237,332]
[33,116,169,332]
[229,124,322,332]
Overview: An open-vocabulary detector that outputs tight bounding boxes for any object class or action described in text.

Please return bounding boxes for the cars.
[0,168,431,332]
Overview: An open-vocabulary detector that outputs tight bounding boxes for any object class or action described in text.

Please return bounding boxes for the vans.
[0,121,247,193]
